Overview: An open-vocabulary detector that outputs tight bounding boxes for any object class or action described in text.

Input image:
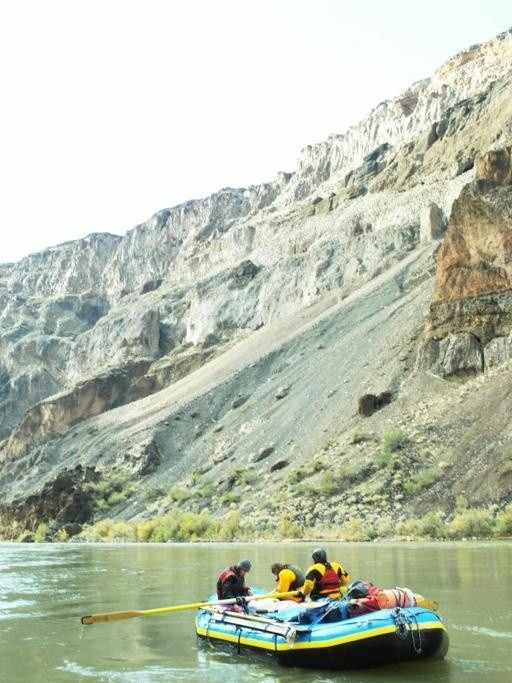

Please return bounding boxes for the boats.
[194,586,450,671]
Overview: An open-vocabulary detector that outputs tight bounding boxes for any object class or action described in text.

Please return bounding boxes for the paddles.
[82,591,303,625]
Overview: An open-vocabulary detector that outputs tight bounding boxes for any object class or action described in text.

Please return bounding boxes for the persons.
[296,550,349,601]
[272,563,306,602]
[217,560,253,613]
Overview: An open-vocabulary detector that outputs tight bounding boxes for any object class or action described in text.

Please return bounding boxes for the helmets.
[238,560,251,572]
[271,563,284,575]
[311,548,327,563]
